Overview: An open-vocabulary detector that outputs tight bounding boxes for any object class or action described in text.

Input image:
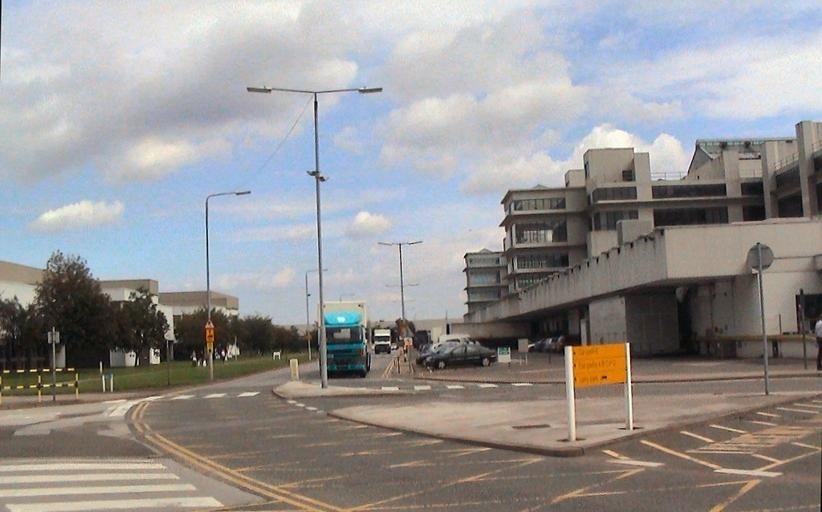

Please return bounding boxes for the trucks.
[314,298,373,380]
[372,326,393,355]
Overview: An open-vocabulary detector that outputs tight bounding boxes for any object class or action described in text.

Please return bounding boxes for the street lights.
[374,237,424,326]
[203,190,253,382]
[242,82,385,390]
[304,268,329,361]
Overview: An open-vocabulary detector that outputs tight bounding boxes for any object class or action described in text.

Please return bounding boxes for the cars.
[390,342,398,351]
[414,342,498,371]
[522,331,581,355]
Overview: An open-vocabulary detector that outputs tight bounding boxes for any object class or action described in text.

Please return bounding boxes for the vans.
[436,333,482,345]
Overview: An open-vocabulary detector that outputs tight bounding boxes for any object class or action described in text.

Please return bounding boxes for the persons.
[813,313,822,372]
